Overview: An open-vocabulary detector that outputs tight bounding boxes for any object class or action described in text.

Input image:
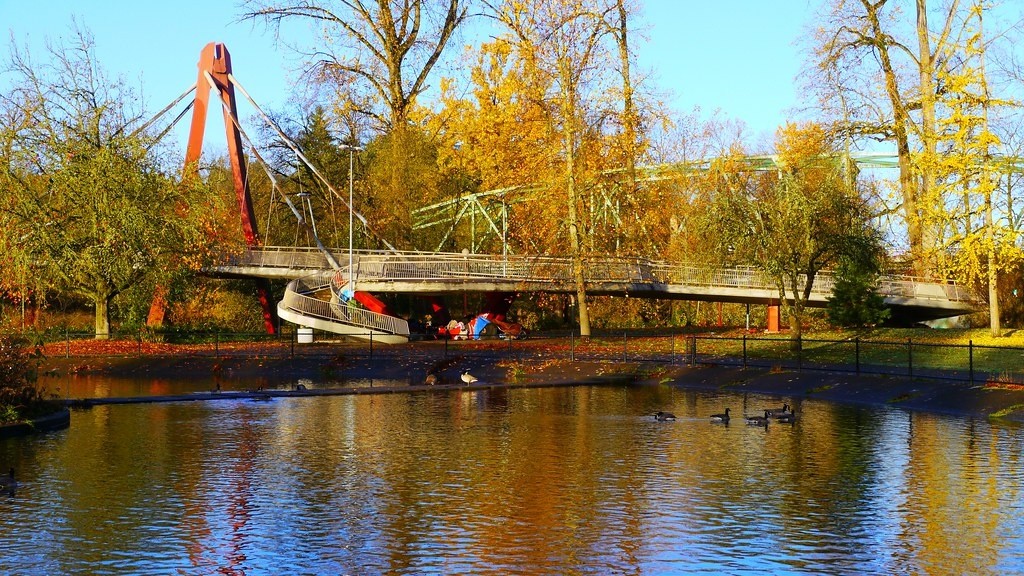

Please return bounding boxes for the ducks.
[746,404,795,424]
[710,408,731,421]
[655,412,677,422]
